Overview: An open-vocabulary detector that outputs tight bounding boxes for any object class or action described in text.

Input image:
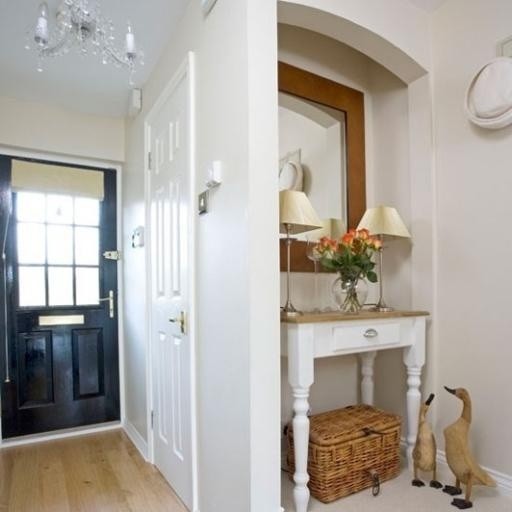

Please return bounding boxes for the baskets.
[285,403,406,506]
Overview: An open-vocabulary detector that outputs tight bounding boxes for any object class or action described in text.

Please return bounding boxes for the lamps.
[278,185,325,319]
[18,0,147,86]
[357,205,415,313]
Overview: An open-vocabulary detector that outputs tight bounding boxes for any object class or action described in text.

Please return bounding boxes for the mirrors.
[277,59,368,275]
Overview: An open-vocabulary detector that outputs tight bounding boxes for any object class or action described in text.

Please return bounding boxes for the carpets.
[278,442,511,512]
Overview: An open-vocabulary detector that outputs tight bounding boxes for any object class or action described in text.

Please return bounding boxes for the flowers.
[308,223,387,312]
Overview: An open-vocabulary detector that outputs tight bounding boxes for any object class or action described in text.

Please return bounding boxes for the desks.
[282,307,432,512]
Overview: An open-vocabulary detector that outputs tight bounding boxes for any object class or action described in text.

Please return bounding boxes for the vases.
[331,276,372,315]
